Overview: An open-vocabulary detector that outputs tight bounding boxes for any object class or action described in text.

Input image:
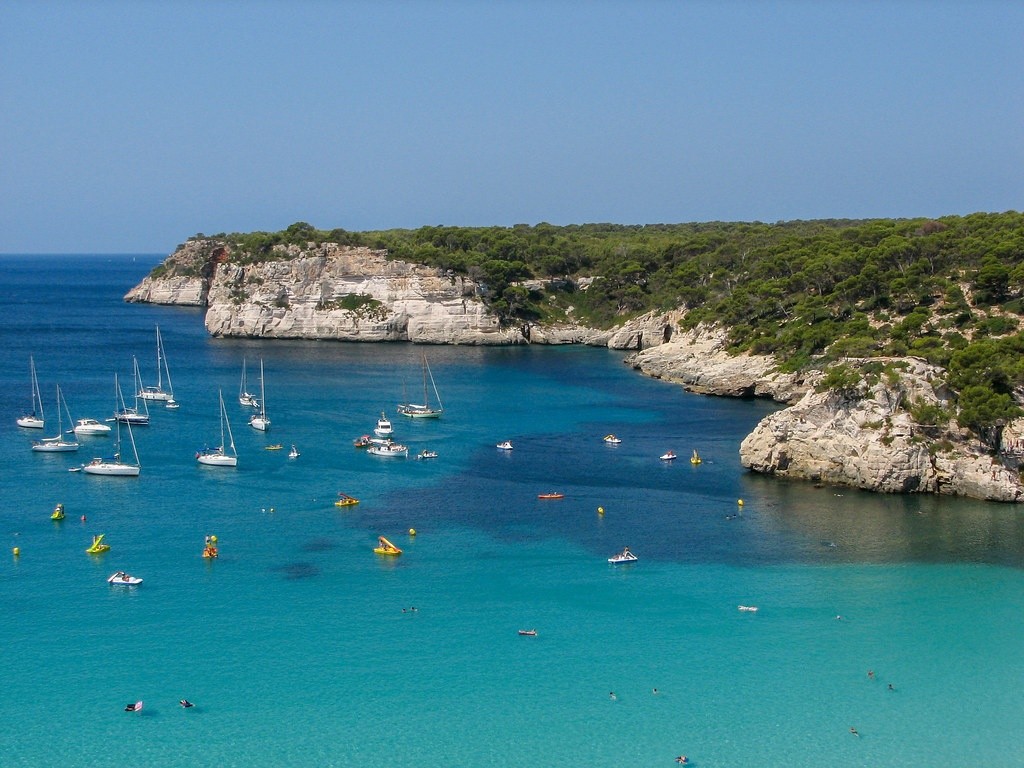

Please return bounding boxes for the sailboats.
[84,371,141,477]
[195,389,238,467]
[16,354,46,428]
[238,357,261,409]
[114,354,151,426]
[134,324,173,401]
[248,358,272,431]
[397,353,444,419]
[31,384,80,452]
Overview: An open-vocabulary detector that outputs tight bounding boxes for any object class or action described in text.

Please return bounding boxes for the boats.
[604,434,622,443]
[497,440,513,450]
[422,452,438,458]
[659,454,676,460]
[68,467,81,472]
[126,700,143,716]
[374,411,394,439]
[108,573,143,585]
[373,536,403,554]
[607,551,638,564]
[539,494,565,498]
[335,494,359,506]
[691,450,702,464]
[354,434,409,457]
[50,504,66,520]
[87,533,110,553]
[74,419,112,435]
[518,628,536,635]
